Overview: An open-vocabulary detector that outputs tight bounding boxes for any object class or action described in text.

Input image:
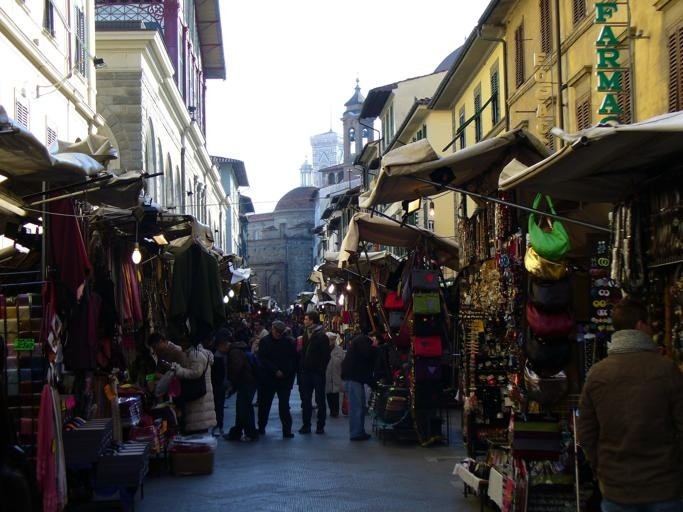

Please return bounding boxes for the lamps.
[0,187,27,218]
[400,196,421,226]
[37,47,105,97]
[153,234,170,246]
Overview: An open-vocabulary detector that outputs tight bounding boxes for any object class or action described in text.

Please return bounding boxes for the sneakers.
[211,424,270,444]
[282,431,295,440]
[297,424,325,434]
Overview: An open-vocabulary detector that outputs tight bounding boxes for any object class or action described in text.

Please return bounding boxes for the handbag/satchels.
[527,273,576,316]
[411,290,442,315]
[180,353,212,402]
[510,357,570,462]
[525,304,576,340]
[411,235,441,292]
[389,310,407,328]
[524,337,570,377]
[527,192,573,261]
[383,290,406,310]
[522,245,569,280]
[412,314,443,337]
[412,335,445,357]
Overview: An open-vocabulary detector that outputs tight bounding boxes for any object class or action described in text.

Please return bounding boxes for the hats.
[271,319,286,332]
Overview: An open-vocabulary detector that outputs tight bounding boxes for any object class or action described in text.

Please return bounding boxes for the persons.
[139,297,400,444]
[574,297,683,511]
